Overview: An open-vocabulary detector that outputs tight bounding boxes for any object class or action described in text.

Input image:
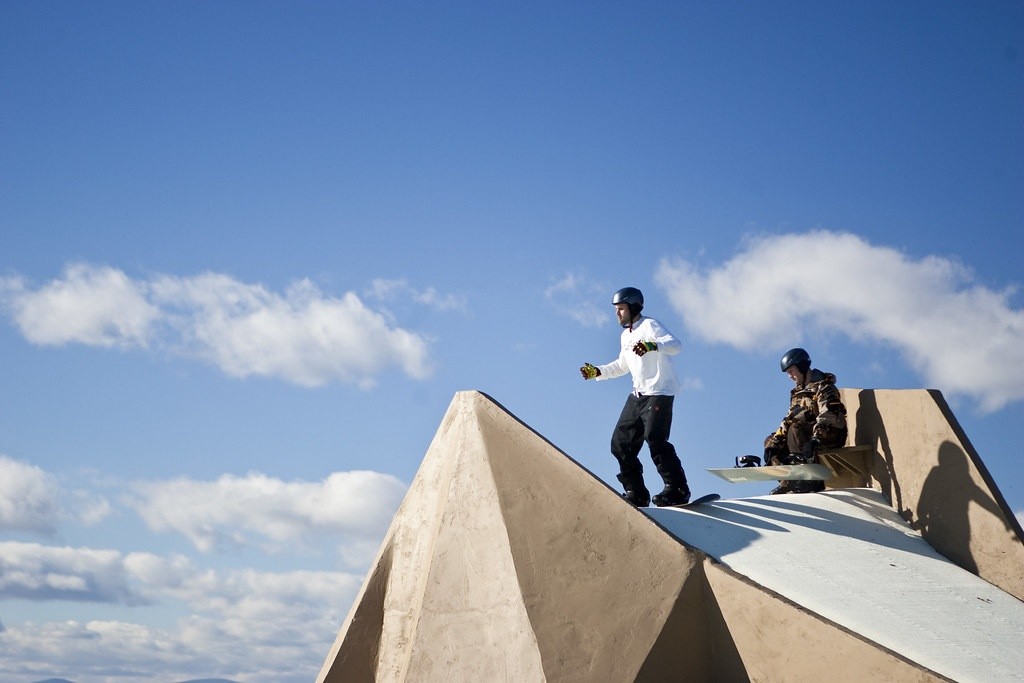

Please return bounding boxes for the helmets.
[612,287,644,306]
[781,348,811,373]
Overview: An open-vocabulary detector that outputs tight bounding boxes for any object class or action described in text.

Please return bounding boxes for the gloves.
[766,446,778,466]
[804,438,822,459]
[580,363,601,380]
[632,340,657,356]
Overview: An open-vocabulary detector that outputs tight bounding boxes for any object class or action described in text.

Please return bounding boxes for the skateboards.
[704,462,833,484]
[675,493,721,508]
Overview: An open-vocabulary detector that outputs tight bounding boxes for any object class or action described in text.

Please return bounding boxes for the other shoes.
[651,483,691,507]
[622,487,651,507]
[796,480,826,493]
[769,484,793,495]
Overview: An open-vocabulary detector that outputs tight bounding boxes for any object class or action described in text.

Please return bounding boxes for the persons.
[763,347,848,495]
[580,287,691,507]
